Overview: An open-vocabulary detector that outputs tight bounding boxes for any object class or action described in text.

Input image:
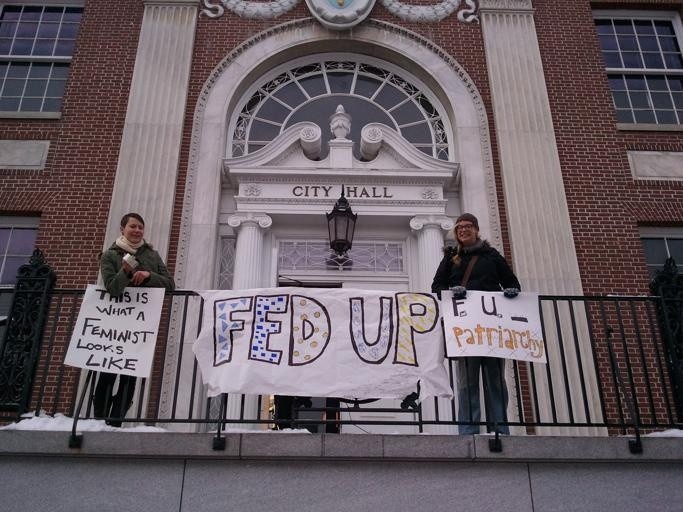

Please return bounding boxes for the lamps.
[321,193,357,269]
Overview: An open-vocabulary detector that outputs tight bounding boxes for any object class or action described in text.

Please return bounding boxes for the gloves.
[450,286,467,299]
[504,287,519,298]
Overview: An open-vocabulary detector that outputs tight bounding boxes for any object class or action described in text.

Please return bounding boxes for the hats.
[456,213,478,227]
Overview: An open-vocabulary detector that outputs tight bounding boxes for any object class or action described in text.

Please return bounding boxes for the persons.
[431,213,520,436]
[95,213,174,427]
